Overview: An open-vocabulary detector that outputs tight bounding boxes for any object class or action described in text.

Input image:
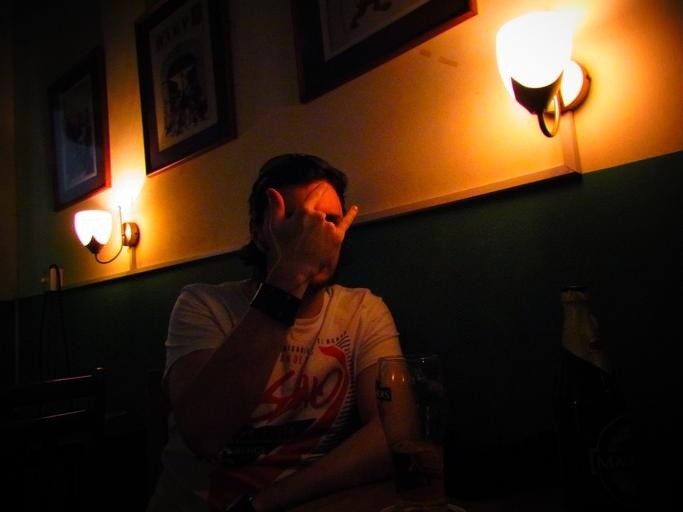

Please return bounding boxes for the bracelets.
[246,284,305,330]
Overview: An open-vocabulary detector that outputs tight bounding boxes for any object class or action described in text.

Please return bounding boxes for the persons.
[146,150,424,512]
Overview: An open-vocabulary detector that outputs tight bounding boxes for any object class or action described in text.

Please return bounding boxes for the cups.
[377,352,451,511]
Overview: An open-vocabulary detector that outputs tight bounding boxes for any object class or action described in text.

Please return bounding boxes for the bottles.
[38,265,68,381]
[552,281,635,511]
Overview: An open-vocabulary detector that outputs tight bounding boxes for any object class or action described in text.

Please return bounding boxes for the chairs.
[0,372,111,511]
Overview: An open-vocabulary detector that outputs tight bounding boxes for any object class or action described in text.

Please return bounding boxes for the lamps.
[495,10,593,138]
[72,209,142,265]
